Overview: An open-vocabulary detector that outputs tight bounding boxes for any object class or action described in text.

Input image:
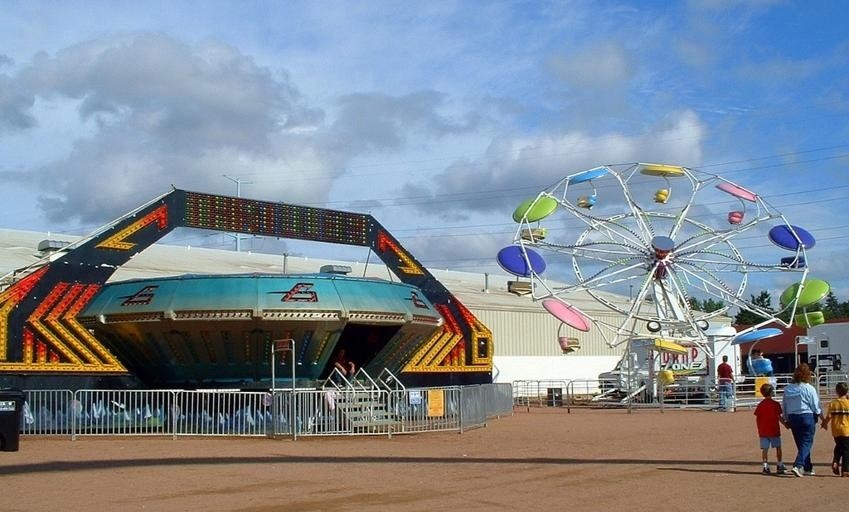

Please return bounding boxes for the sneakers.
[803,470,815,475]
[762,467,771,475]
[791,467,802,477]
[832,463,849,477]
[776,464,788,474]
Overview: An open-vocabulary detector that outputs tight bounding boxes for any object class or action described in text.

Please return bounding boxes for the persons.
[717,355,734,407]
[754,382,790,475]
[750,348,756,359]
[783,362,828,478]
[819,381,849,477]
[755,349,764,359]
[331,347,357,389]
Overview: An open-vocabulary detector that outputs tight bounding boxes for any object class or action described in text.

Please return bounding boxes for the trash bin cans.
[548,388,562,406]
[0,389,25,452]
